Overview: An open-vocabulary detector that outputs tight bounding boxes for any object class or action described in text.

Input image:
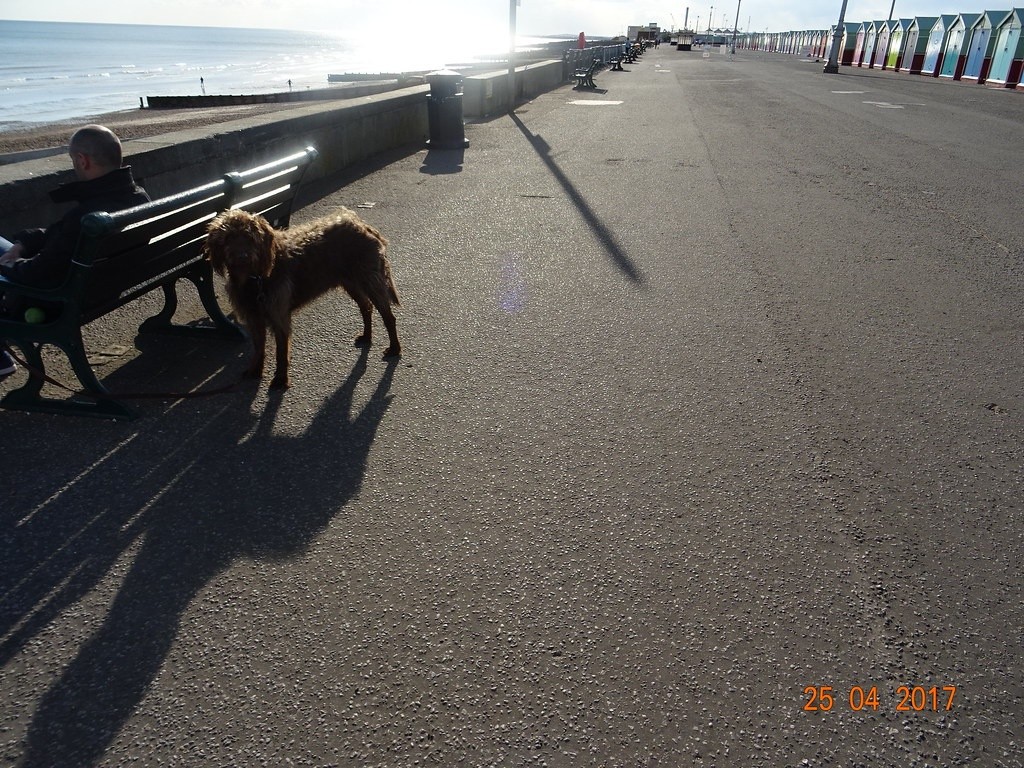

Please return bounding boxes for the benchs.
[568,59,601,91]
[606,45,647,71]
[0,146,318,420]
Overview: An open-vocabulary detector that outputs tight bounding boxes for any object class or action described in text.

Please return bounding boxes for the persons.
[626,37,631,54]
[200,76,204,88]
[0,125,152,374]
[654,37,662,50]
[638,35,646,54]
[285,79,293,88]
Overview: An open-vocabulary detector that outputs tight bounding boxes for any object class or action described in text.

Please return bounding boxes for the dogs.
[205,208,402,394]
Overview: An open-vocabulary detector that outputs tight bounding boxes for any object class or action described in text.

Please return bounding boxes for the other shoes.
[0,351,18,375]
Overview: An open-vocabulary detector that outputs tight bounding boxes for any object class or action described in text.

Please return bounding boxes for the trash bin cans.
[425,70,469,150]
